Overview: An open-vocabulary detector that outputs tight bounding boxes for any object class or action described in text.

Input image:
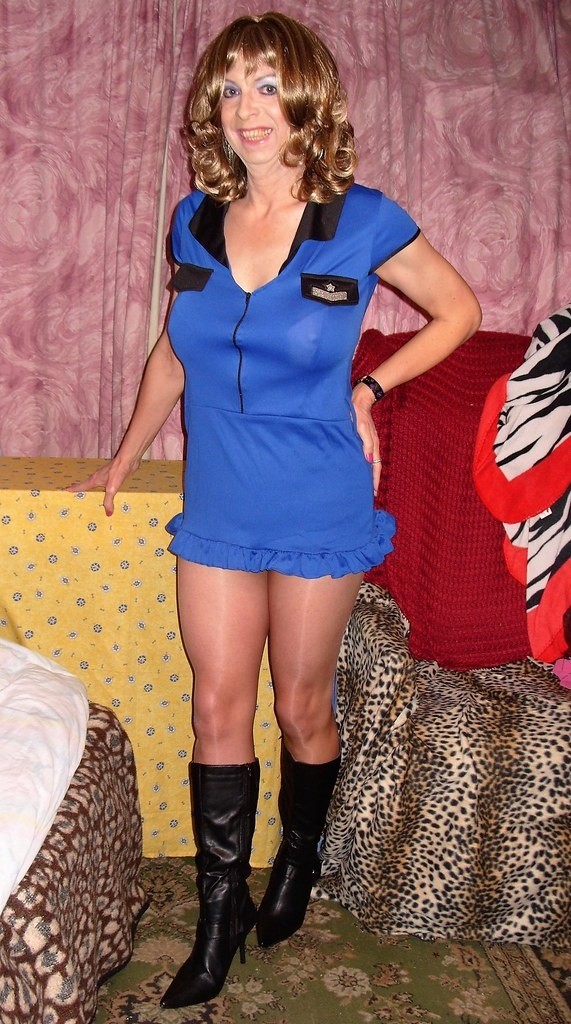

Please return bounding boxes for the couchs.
[308,581,571,953]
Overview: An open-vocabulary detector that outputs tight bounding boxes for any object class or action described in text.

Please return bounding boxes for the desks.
[0,457,281,868]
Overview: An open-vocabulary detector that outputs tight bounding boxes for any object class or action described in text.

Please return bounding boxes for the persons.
[64,12,483,1008]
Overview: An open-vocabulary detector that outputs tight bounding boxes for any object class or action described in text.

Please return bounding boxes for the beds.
[0,639,146,1024]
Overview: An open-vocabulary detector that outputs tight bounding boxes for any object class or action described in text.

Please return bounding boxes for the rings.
[372,459,382,463]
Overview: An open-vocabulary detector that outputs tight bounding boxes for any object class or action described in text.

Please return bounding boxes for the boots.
[256,736,342,946]
[160,758,261,1009]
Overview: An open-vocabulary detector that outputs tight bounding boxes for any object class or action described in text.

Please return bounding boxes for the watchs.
[354,375,385,405]
